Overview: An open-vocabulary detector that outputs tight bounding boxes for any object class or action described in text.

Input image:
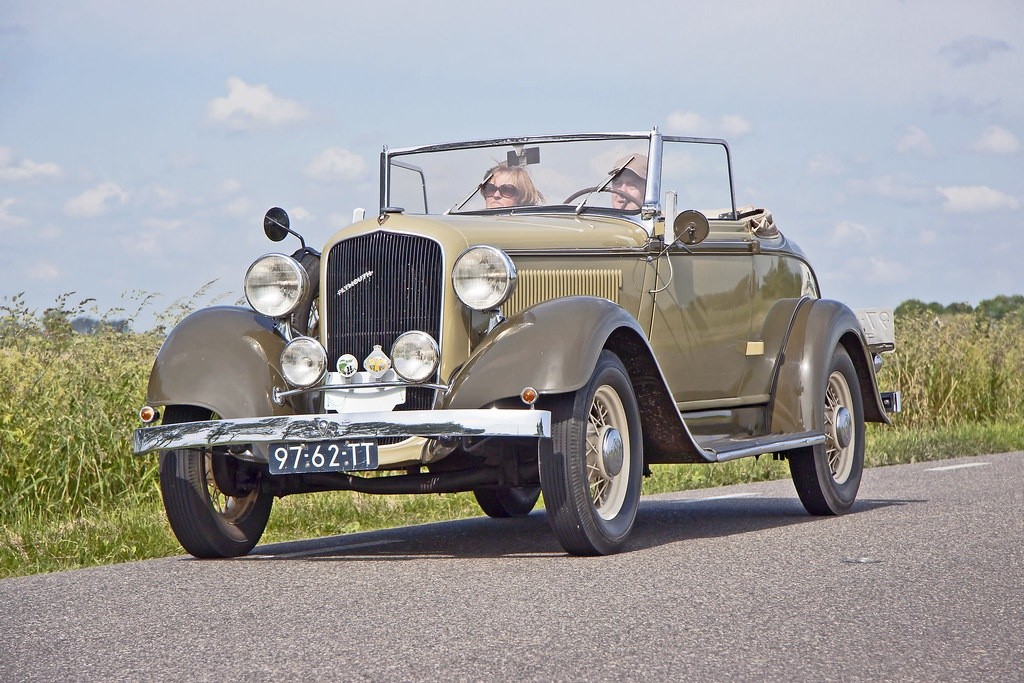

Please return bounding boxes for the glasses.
[480,182,520,198]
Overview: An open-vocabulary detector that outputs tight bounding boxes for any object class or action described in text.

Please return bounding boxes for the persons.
[608,153,647,210]
[482,163,540,207]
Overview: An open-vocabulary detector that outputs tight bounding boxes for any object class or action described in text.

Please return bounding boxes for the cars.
[130,127,903,558]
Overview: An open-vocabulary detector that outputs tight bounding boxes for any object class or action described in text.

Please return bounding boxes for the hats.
[608,152,647,181]
